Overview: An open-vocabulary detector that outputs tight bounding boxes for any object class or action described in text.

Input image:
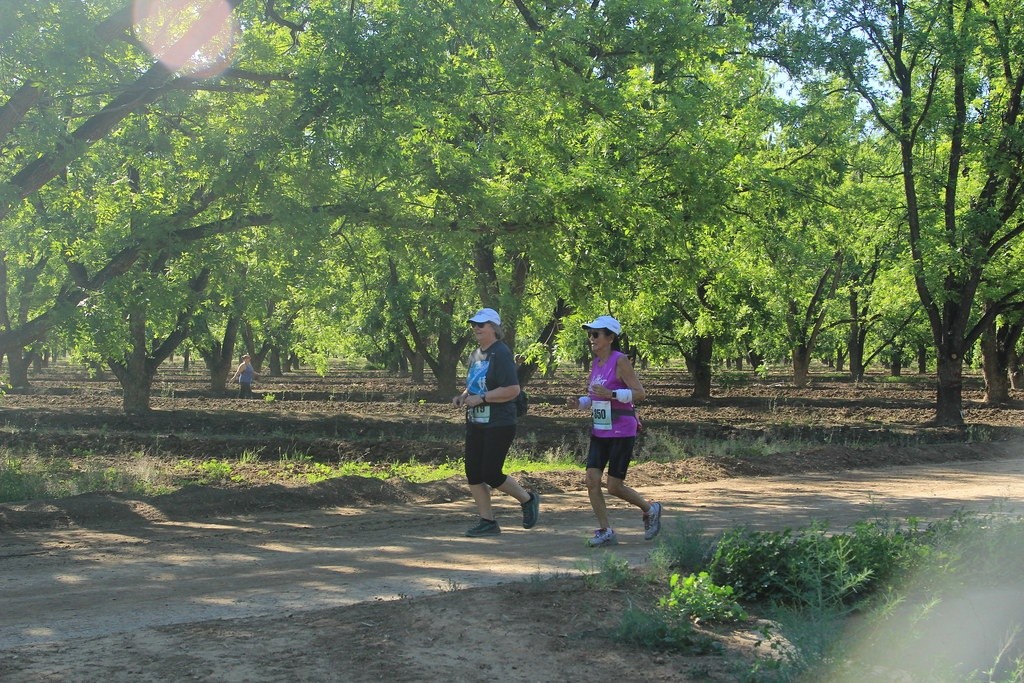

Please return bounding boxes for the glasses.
[588,331,606,338]
[471,322,485,328]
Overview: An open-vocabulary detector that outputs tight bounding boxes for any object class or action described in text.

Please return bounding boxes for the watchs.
[480,392,486,403]
[611,390,618,400]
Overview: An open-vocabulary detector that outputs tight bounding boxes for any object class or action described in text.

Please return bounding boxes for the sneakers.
[520,491,539,529]
[465,518,501,537]
[586,527,618,546]
[642,502,662,539]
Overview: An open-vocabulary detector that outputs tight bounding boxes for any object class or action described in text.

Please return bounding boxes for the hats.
[581,316,620,335]
[467,307,500,326]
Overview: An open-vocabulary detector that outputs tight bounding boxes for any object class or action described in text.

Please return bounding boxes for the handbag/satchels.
[515,390,528,416]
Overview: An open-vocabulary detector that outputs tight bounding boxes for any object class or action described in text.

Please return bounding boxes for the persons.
[566,316,662,548]
[231,354,258,399]
[452,307,539,537]
[514,354,526,366]
